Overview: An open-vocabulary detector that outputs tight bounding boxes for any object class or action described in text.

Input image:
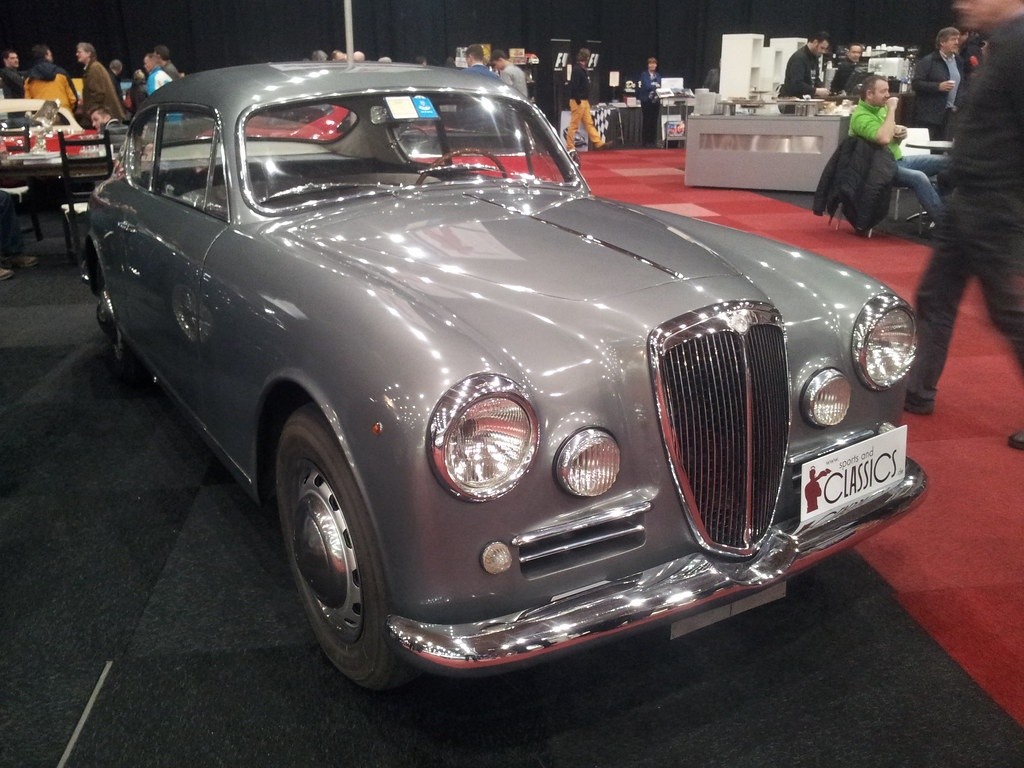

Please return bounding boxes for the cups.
[806,105,816,117]
[823,102,836,114]
[894,125,903,138]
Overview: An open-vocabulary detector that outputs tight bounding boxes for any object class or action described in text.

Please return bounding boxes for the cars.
[0,97,107,158]
[198,93,559,180]
[78,63,930,692]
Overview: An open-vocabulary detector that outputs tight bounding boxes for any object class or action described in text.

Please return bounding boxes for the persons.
[311,49,392,62]
[90,106,112,132]
[460,44,498,80]
[641,57,661,147]
[902,0,1024,449]
[24,45,79,121]
[415,56,426,65]
[778,31,831,114]
[75,43,125,124]
[567,49,613,150]
[912,24,987,141]
[847,74,947,222]
[110,59,126,103]
[490,49,527,98]
[703,69,719,93]
[0,190,38,281]
[131,46,182,123]
[0,51,25,127]
[830,43,862,95]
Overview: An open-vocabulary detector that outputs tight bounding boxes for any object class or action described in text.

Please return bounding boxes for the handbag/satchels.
[124,89,135,113]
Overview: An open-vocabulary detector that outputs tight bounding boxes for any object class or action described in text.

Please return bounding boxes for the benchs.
[140,151,384,199]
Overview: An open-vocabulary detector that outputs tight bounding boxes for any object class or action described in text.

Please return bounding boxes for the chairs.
[0,123,43,242]
[812,135,902,237]
[57,130,120,263]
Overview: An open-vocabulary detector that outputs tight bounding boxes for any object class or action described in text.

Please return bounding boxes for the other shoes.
[598,141,613,151]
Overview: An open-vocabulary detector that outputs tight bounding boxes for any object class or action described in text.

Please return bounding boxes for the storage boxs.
[623,96,641,107]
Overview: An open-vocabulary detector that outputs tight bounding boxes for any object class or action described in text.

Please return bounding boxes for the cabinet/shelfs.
[719,33,809,102]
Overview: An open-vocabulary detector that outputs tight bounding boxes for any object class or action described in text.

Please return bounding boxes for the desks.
[0,126,97,156]
[905,139,958,230]
[656,100,698,149]
[560,105,643,152]
[0,148,118,178]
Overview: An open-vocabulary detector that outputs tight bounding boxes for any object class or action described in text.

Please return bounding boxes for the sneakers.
[904,390,934,417]
[0,268,15,281]
[1008,430,1024,449]
[7,254,38,268]
